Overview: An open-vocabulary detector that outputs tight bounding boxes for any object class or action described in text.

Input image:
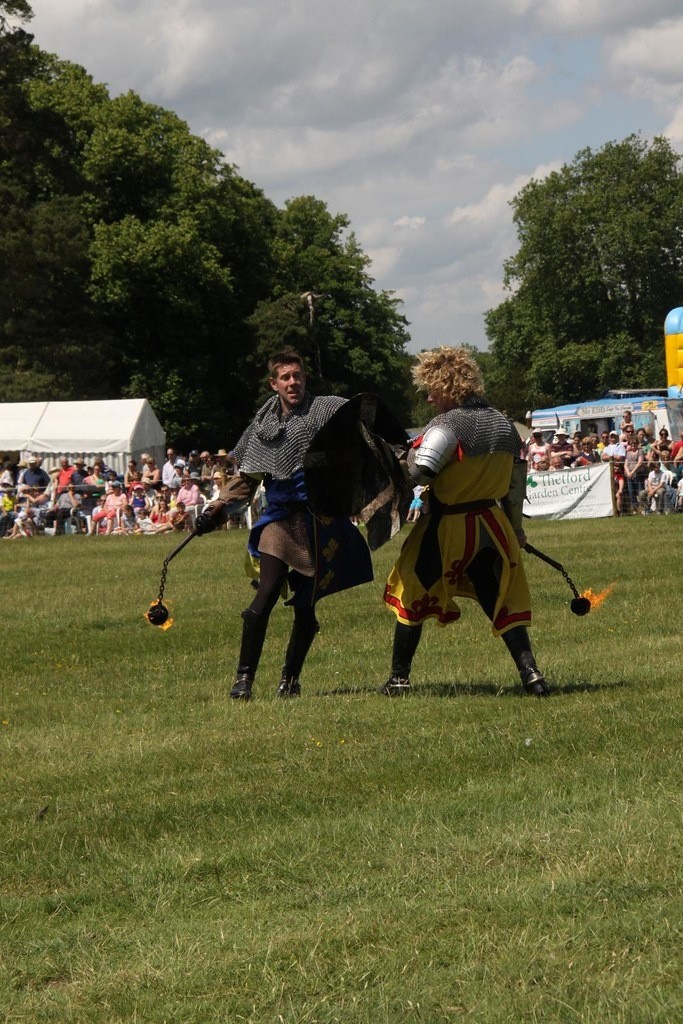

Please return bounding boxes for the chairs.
[96,509,121,536]
[190,494,207,529]
[63,508,82,534]
[644,472,672,511]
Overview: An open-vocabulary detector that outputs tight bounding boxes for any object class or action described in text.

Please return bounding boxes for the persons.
[0,449,269,535]
[376,347,551,698]
[191,350,415,701]
[402,410,683,527]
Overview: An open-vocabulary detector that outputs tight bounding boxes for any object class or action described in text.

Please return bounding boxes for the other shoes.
[274,620,320,697]
[379,678,410,698]
[524,674,548,695]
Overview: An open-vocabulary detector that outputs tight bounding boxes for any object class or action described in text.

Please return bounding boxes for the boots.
[230,608,264,698]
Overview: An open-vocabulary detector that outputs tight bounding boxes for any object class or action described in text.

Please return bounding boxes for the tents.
[0,399,166,477]
[532,396,683,444]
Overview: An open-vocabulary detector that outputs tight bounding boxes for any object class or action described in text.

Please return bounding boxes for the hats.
[174,462,184,468]
[215,449,227,456]
[532,454,542,464]
[190,450,197,456]
[553,428,568,435]
[213,472,224,479]
[134,485,144,490]
[29,456,37,464]
[74,458,86,467]
[49,468,60,474]
[17,461,29,468]
[532,428,542,434]
[610,430,619,437]
[111,481,123,489]
[190,471,201,481]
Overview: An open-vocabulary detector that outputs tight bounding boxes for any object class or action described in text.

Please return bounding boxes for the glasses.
[534,436,542,439]
[602,436,608,439]
[68,487,76,489]
[660,434,668,437]
[626,431,634,434]
[156,496,163,499]
[161,489,169,493]
